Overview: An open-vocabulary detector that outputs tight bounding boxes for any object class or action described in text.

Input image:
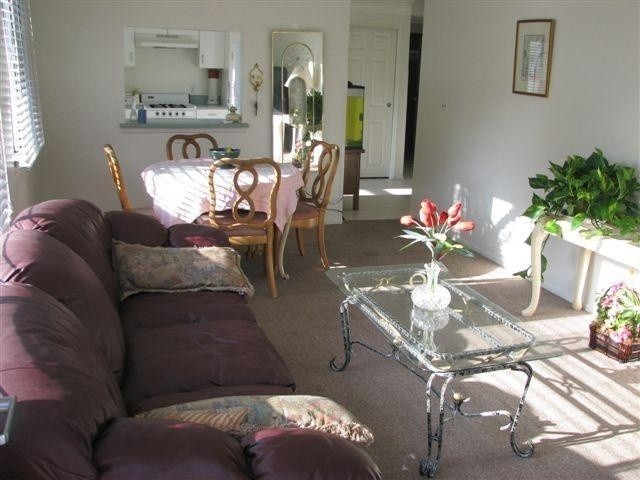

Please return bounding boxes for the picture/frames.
[511,18,554,96]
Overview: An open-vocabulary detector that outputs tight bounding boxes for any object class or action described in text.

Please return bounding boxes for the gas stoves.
[143,104,197,118]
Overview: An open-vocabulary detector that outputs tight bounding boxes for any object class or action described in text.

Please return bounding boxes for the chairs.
[103,140,158,219]
[196,157,281,299]
[289,140,337,268]
[165,133,219,158]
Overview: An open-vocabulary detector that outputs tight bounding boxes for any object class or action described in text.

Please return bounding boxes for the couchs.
[0,197,382,478]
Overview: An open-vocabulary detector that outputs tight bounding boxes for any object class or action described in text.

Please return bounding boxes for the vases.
[411,262,451,309]
[588,324,639,363]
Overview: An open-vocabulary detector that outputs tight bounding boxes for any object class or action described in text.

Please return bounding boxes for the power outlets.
[438,99,448,113]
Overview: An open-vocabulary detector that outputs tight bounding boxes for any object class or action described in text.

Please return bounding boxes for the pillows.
[134,394,373,450]
[113,238,257,301]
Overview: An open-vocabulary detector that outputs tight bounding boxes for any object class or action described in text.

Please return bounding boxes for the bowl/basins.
[208,148,240,169]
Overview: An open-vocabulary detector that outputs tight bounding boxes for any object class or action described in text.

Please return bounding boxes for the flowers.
[394,199,471,263]
[594,284,640,340]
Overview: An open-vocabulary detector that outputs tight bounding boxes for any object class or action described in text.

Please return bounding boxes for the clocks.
[271,31,325,162]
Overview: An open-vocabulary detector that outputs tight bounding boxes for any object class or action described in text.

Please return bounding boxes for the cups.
[138,110,146,123]
[125,109,132,119]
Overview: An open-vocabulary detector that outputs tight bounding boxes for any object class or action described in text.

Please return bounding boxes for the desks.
[519,206,640,319]
[344,148,366,213]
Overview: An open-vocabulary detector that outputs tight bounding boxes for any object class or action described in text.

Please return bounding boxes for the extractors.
[134,34,199,50]
